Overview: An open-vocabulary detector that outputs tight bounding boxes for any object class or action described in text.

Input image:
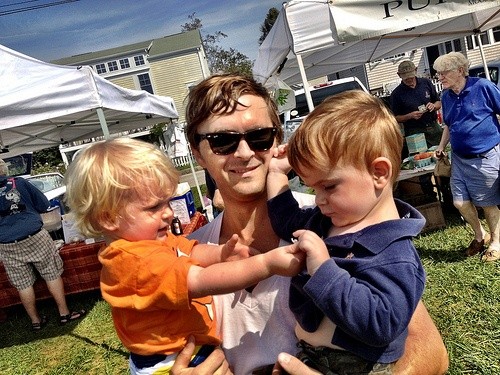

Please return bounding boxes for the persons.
[433,55,500,262]
[245,89,426,375]
[448,52,471,78]
[0,158,85,329]
[170,73,448,375]
[388,60,464,226]
[203,168,225,218]
[63,136,305,375]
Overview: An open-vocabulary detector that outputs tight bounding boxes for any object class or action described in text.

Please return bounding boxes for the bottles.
[173,216,184,235]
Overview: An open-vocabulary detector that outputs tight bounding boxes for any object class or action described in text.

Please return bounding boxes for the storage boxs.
[170,182,195,217]
[404,195,446,231]
[406,133,428,155]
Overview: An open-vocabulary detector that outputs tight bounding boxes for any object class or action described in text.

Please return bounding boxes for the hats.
[397,61,416,80]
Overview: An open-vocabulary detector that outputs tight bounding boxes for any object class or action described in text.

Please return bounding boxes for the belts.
[0,228,41,244]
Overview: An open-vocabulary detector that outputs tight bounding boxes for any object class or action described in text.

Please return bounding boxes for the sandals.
[32,315,48,331]
[61,309,86,323]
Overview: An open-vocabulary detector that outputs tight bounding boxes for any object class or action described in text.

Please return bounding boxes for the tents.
[0,40,206,211]
[252,0,500,114]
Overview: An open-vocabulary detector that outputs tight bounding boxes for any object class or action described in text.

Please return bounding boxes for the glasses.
[436,70,450,77]
[194,125,277,155]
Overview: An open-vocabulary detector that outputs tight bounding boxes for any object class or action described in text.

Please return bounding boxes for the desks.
[0,214,202,308]
[394,159,454,230]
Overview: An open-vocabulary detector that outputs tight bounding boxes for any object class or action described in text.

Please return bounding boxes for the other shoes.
[482,245,500,263]
[462,232,491,257]
